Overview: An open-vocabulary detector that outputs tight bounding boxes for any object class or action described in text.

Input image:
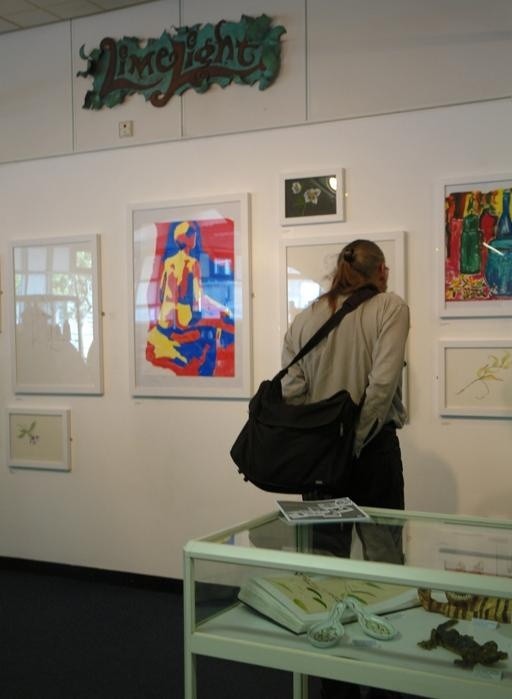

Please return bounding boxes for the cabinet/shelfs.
[180,506,511,699]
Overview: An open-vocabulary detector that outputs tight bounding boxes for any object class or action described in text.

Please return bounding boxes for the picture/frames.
[4,408,72,473]
[6,231,104,396]
[435,173,512,319]
[279,229,409,426]
[440,339,512,417]
[128,192,255,400]
[278,168,345,225]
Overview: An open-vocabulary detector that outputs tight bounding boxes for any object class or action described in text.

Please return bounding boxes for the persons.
[275,237,411,565]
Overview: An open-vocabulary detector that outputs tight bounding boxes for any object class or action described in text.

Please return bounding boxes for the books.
[236,572,421,636]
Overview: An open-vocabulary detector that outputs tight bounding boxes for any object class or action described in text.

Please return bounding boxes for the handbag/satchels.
[230,380,358,493]
